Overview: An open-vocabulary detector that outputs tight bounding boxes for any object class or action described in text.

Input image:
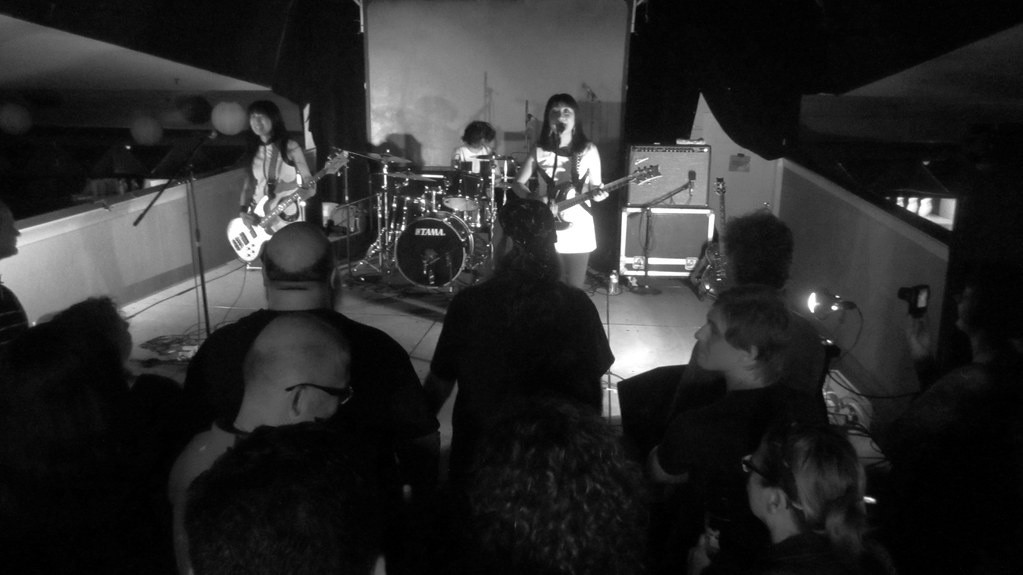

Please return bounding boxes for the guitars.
[226,148,351,263]
[534,163,662,231]
[698,177,734,300]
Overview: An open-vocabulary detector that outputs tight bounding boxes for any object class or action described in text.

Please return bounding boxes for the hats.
[497,199,557,244]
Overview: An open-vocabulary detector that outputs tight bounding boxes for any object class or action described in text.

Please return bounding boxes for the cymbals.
[467,153,516,162]
[491,181,515,191]
[367,151,413,166]
[373,170,439,183]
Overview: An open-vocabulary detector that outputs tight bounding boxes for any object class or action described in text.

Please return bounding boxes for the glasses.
[285,383,354,405]
[741,454,793,501]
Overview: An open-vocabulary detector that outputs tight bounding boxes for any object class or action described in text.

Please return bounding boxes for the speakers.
[625,142,711,208]
[617,364,689,440]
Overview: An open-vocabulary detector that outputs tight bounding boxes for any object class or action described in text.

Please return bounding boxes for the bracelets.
[239,205,247,212]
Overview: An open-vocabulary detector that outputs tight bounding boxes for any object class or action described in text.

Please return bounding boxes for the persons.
[453,121,503,179]
[0,202,445,575]
[645,213,828,574]
[513,95,612,290]
[453,396,645,575]
[877,263,1022,575]
[239,98,317,301]
[689,418,897,575]
[421,199,614,475]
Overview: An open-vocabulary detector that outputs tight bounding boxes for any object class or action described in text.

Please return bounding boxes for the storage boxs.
[620,206,716,288]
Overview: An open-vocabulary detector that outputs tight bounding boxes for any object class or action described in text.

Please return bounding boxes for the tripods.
[352,162,402,273]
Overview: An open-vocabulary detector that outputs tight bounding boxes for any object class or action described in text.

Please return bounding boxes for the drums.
[385,194,433,239]
[441,168,485,213]
[451,194,493,230]
[392,210,475,289]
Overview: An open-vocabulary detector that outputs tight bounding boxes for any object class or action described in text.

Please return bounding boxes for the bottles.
[609,269,618,294]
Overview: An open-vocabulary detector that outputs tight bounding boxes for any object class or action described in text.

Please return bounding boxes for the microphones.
[200,130,217,146]
[556,123,565,136]
[688,170,696,196]
[422,256,429,274]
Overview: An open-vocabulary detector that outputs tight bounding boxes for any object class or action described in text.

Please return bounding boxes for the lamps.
[897,285,930,320]
[802,285,858,322]
